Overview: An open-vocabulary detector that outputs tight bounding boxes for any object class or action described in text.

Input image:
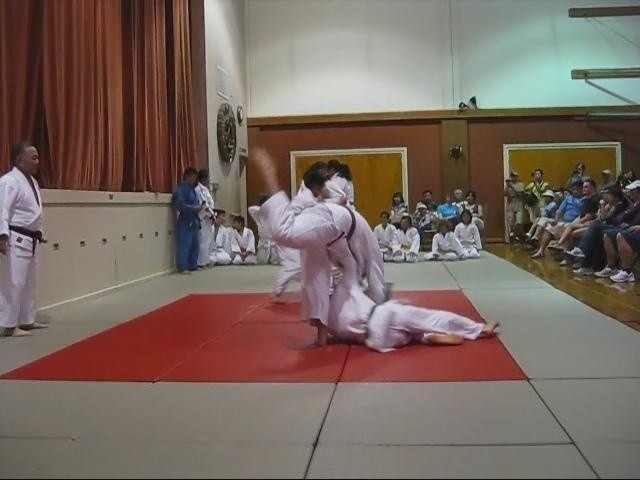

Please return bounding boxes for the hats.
[510,168,520,177]
[543,189,556,199]
[624,180,640,191]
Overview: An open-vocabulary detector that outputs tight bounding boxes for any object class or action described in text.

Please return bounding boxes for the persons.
[260,160,386,352]
[172,167,216,274]
[249,193,282,265]
[208,209,257,265]
[374,189,484,263]
[0,142,49,337]
[328,256,500,352]
[502,162,639,283]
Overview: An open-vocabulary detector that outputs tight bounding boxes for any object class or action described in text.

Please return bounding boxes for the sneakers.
[525,232,546,259]
[566,247,585,260]
[609,269,636,284]
[547,243,564,253]
[594,267,620,279]
[572,267,594,277]
